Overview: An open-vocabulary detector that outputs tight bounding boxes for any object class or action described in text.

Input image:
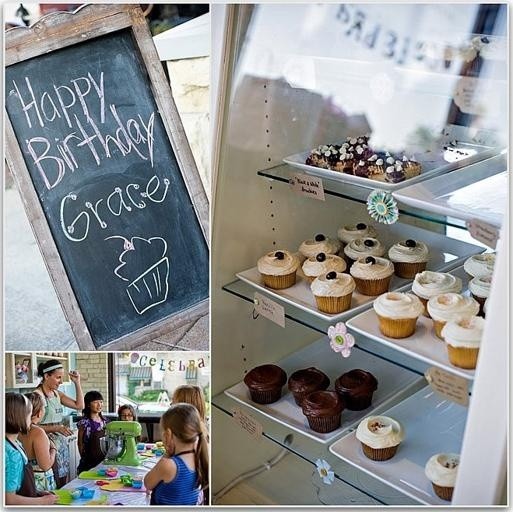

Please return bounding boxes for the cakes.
[445,36,507,74]
[355,415,403,461]
[243,364,377,433]
[256,223,494,368]
[425,453,460,500]
[307,136,421,184]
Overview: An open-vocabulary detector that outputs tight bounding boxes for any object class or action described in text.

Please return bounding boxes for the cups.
[145,442,163,456]
[255,221,497,370]
[97,467,142,489]
[69,486,95,501]
[239,360,464,502]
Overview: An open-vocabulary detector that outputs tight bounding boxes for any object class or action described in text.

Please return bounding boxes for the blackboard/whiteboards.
[4,4,215,351]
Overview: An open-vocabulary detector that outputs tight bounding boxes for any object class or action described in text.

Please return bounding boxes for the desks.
[56,443,166,505]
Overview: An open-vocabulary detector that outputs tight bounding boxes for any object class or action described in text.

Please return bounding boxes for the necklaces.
[176,449,196,455]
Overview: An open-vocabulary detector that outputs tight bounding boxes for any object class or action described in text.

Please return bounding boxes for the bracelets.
[49,448,57,451]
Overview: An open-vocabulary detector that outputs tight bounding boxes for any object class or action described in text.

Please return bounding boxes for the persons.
[5,392,58,504]
[19,390,57,491]
[169,384,205,505]
[117,404,137,421]
[76,391,111,476]
[33,359,85,489]
[143,403,208,505]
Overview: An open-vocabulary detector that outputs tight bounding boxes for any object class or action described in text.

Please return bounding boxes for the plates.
[388,152,509,233]
[326,380,472,503]
[223,334,426,445]
[233,217,488,320]
[280,133,499,194]
[344,251,496,377]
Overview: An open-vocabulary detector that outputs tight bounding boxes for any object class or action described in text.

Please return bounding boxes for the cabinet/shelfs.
[212,4,507,505]
[5,353,71,389]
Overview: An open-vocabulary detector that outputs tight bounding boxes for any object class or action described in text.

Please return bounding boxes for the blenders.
[98,419,142,467]
